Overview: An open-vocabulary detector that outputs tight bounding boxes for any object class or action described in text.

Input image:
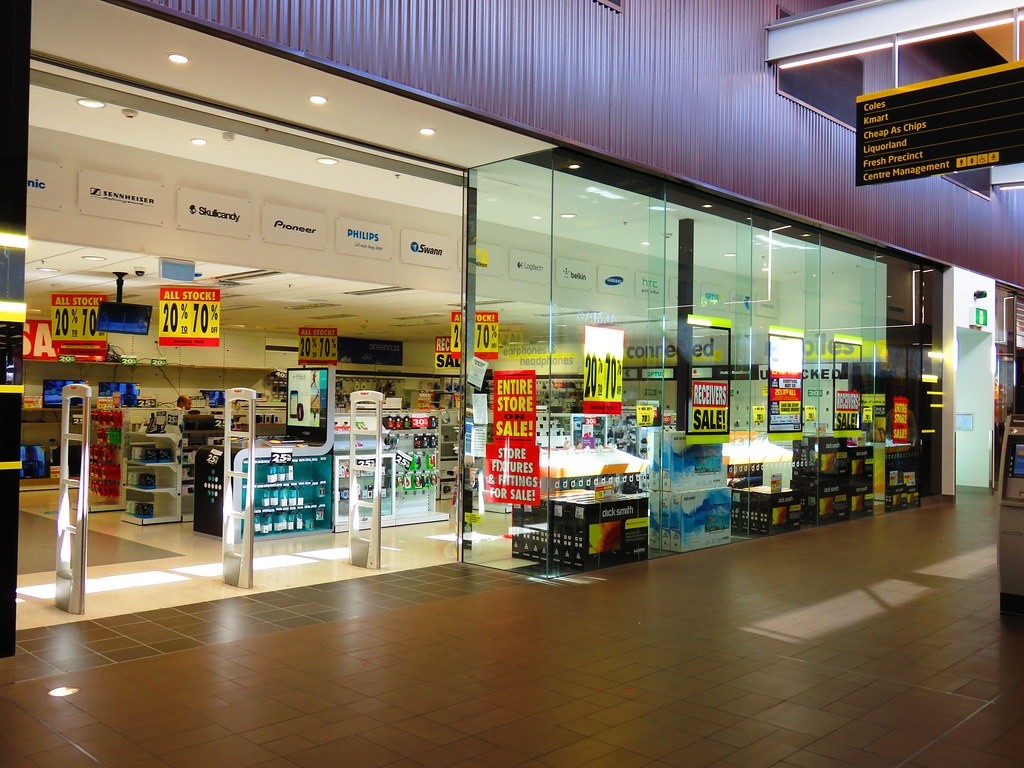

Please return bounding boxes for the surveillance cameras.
[135,266,145,276]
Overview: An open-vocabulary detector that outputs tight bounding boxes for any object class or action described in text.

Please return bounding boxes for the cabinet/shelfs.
[20,358,275,486]
[119,409,183,526]
[436,408,460,500]
[182,414,286,522]
[332,411,449,534]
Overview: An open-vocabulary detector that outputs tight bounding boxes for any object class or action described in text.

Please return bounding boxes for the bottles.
[415,437,420,448]
[297,486,305,505]
[270,487,278,506]
[279,487,288,507]
[423,437,427,448]
[262,489,269,506]
[432,418,436,429]
[289,486,296,506]
[267,461,293,484]
[431,437,435,448]
[388,417,411,429]
[255,510,303,534]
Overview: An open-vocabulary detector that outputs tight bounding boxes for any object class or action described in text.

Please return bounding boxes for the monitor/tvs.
[201,390,226,406]
[42,378,85,408]
[98,382,138,407]
[20,445,50,479]
[95,301,153,335]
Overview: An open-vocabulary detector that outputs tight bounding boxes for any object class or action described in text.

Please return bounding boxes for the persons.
[176,395,192,410]
[310,371,318,388]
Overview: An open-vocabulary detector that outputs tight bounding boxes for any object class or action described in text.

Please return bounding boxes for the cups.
[132,447,174,463]
[126,500,153,519]
[128,471,156,489]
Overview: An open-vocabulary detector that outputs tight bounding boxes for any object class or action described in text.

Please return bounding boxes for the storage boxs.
[97,397,114,409]
[43,379,85,408]
[125,436,224,518]
[23,395,42,408]
[99,381,140,407]
[512,399,922,574]
[20,445,47,479]
[49,466,61,478]
